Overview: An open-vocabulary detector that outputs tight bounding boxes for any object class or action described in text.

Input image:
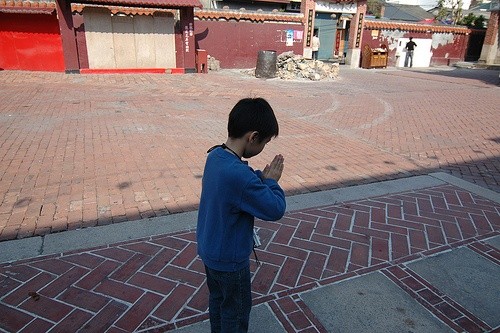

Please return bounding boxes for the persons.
[312,33,320,60]
[197,97,287,333]
[404,37,417,68]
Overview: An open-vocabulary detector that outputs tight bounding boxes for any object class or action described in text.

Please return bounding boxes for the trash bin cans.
[362,44,388,69]
